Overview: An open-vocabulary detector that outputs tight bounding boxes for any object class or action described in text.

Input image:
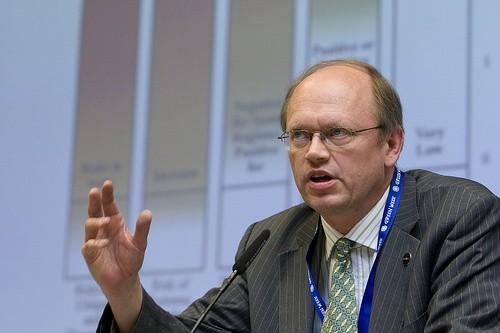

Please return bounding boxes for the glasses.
[278,125,386,151]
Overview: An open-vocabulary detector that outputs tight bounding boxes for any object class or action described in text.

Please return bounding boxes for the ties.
[320,237,359,333]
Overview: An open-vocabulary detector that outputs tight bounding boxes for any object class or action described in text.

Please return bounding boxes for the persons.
[81,59,499,333]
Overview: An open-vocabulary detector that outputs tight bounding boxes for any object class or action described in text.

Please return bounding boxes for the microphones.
[191,228,270,333]
